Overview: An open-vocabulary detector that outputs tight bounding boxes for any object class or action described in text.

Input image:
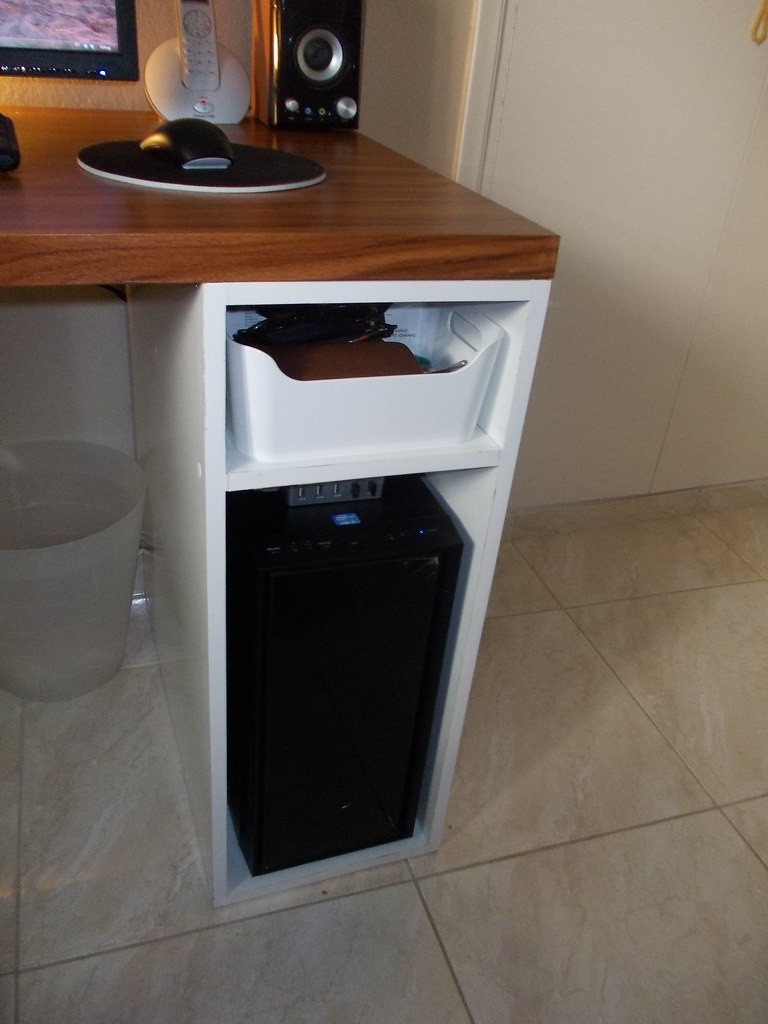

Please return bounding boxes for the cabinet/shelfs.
[119,279,560,908]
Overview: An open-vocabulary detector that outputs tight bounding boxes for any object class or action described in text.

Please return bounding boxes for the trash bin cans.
[1,440,146,700]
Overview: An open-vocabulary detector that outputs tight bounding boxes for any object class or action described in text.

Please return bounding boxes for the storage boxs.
[226,304,507,465]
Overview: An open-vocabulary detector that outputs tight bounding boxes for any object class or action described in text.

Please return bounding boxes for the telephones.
[144,0,251,125]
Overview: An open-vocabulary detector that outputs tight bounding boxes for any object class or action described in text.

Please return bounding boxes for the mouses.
[140,118,236,169]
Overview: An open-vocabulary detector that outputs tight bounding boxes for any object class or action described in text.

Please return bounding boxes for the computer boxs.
[226,476,460,880]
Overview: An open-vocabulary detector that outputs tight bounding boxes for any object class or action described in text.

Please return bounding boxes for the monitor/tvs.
[0,0,139,81]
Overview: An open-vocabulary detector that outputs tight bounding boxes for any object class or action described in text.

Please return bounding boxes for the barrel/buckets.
[0,439,147,703]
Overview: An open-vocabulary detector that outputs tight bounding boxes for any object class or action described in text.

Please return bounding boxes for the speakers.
[252,0,366,129]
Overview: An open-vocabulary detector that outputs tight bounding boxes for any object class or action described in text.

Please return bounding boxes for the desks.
[0,104,559,910]
